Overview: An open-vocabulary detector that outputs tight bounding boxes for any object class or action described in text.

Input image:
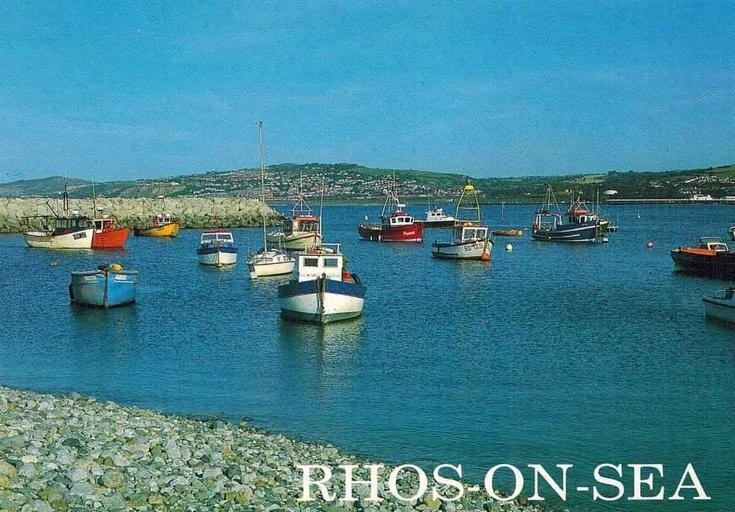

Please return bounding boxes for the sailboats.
[27,177,132,248]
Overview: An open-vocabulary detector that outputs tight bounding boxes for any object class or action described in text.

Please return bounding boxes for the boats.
[671,226,735,278]
[245,121,620,324]
[69,264,140,309]
[196,227,238,267]
[701,285,735,324]
[134,213,180,238]
